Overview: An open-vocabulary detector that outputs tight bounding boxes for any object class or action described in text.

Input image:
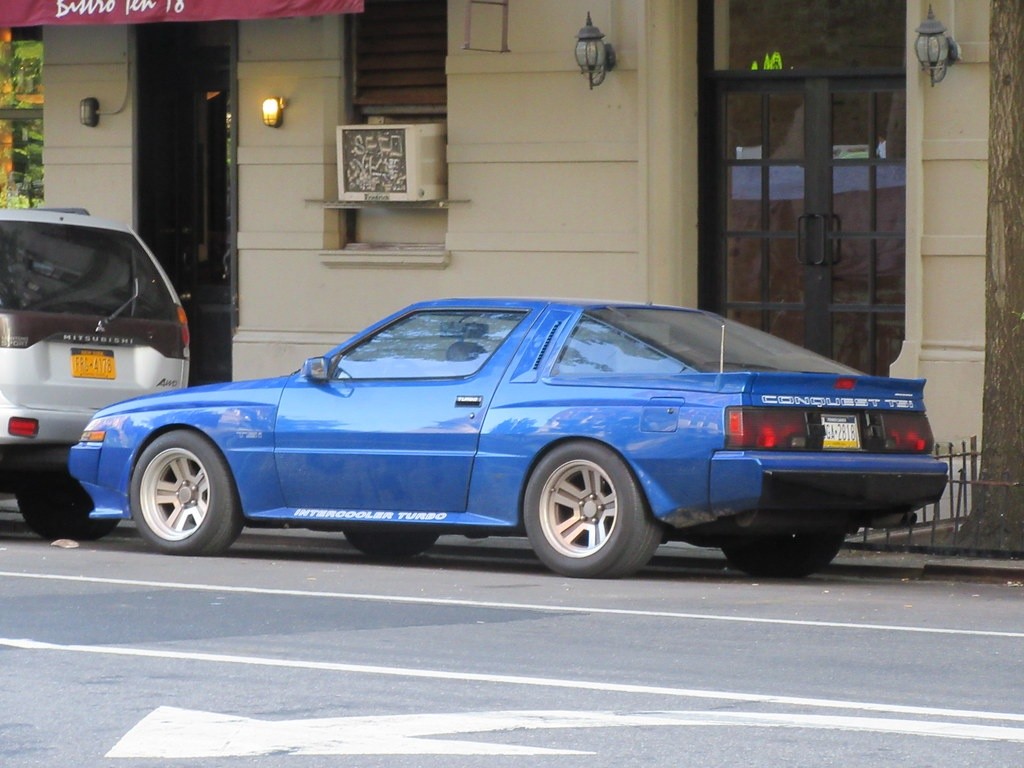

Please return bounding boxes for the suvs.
[0,207,190,541]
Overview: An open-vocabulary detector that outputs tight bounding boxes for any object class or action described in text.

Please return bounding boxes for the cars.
[67,297,948,579]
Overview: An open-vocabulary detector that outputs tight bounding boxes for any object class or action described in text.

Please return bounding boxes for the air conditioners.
[337,121,448,202]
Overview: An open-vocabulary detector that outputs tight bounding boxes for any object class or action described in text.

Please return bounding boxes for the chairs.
[445,341,488,375]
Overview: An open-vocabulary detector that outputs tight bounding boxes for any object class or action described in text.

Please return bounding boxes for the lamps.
[574,9,616,90]
[262,96,285,130]
[78,98,99,127]
[914,4,960,88]
